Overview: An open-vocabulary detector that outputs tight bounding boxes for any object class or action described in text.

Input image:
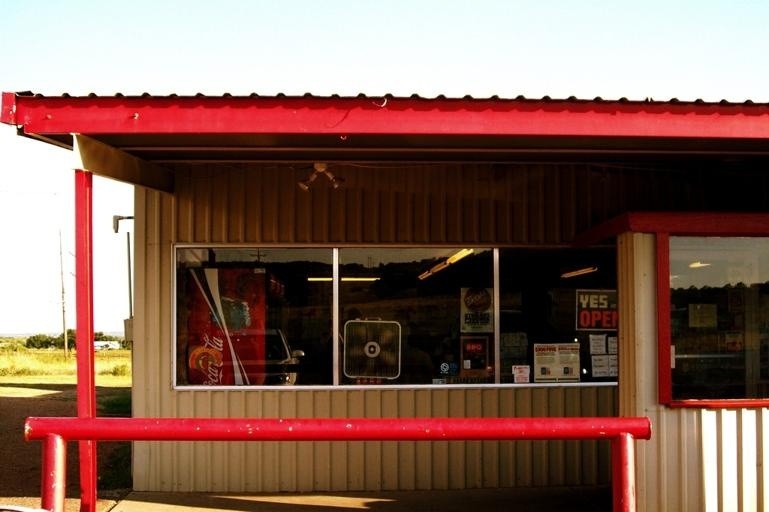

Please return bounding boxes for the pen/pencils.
[93,341,121,354]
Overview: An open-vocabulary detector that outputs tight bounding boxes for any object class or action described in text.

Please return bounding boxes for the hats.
[205,328,309,386]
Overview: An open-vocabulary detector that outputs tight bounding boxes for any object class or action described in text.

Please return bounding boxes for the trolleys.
[343,318,402,380]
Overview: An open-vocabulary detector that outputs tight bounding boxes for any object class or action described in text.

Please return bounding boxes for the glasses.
[418,248,475,280]
[298,165,346,191]
[307,255,380,281]
